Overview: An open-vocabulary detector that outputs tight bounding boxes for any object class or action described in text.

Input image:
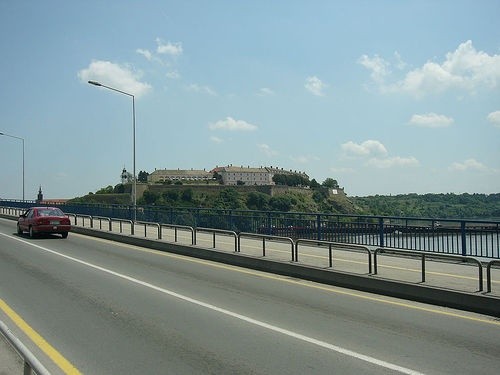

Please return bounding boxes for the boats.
[430,221,443,227]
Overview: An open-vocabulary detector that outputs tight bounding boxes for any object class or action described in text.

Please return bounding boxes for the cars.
[16,207,71,238]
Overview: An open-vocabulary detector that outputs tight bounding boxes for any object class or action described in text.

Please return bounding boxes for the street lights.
[0,132,25,209]
[88,80,136,223]
